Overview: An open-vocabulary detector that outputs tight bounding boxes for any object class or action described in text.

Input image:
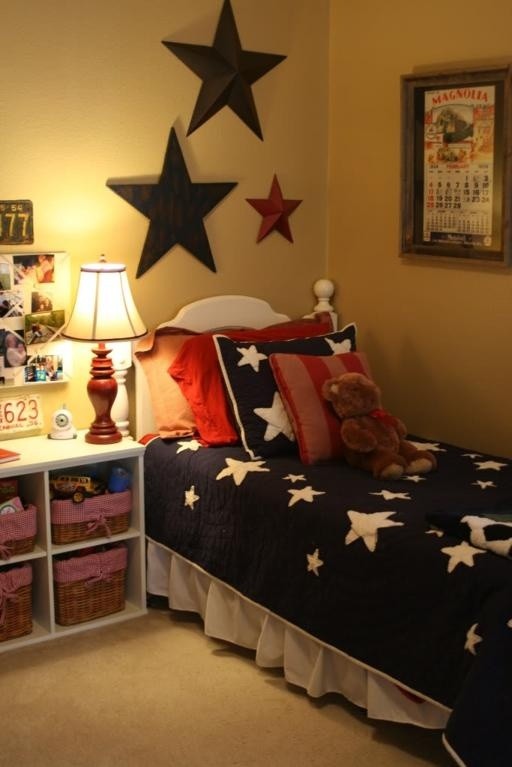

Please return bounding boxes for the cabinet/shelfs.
[0,430,149,653]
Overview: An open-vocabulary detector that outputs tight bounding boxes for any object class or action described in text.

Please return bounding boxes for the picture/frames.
[398,62,511,268]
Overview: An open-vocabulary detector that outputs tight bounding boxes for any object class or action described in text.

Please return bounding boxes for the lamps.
[60,253,149,445]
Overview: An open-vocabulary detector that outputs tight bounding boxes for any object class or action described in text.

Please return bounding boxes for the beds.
[110,279,512,767]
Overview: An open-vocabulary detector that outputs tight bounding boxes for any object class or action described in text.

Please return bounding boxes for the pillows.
[134,312,383,466]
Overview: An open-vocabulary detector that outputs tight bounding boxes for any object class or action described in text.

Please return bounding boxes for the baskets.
[0,504,38,558]
[51,488,132,545]
[1,561,33,641]
[54,543,128,626]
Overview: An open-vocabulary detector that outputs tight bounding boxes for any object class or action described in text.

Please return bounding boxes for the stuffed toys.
[321,371,437,481]
